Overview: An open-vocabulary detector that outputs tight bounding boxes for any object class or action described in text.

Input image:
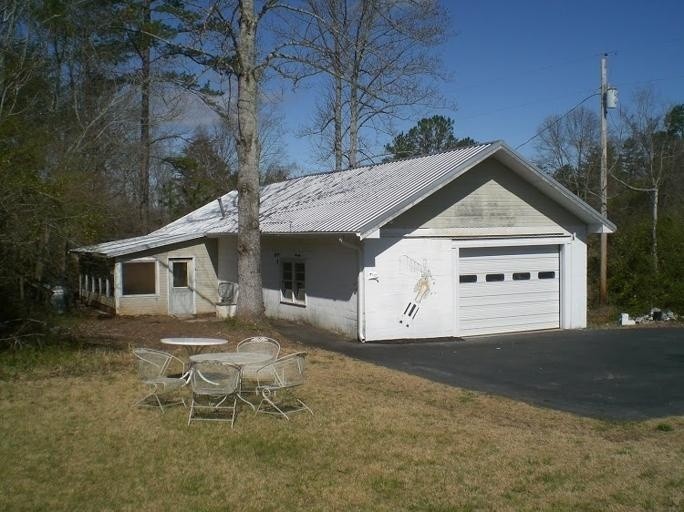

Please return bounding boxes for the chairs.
[132,335,314,430]
